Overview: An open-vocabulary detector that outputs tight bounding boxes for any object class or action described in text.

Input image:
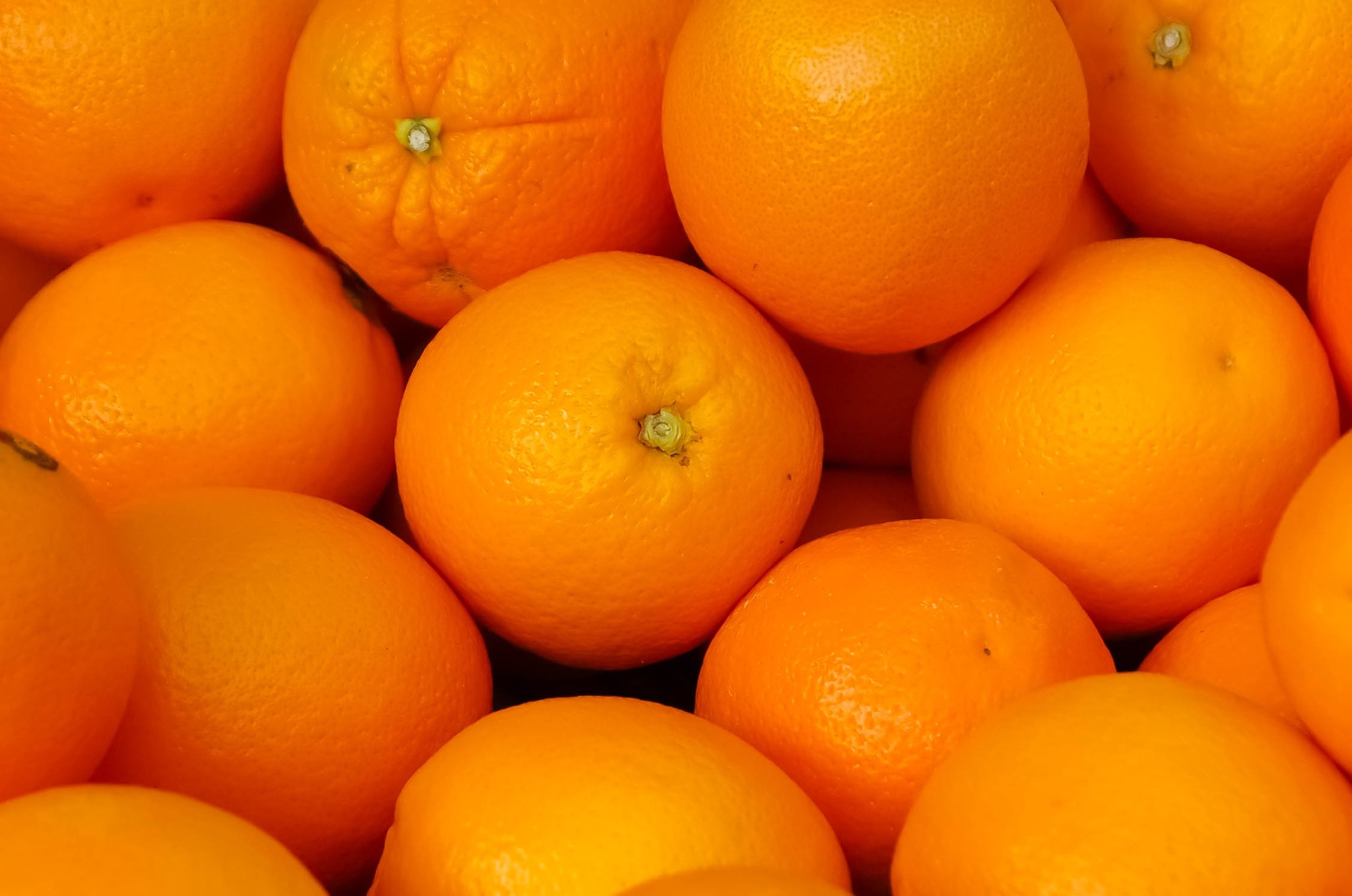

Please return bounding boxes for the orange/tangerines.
[0,0,1352,896]
